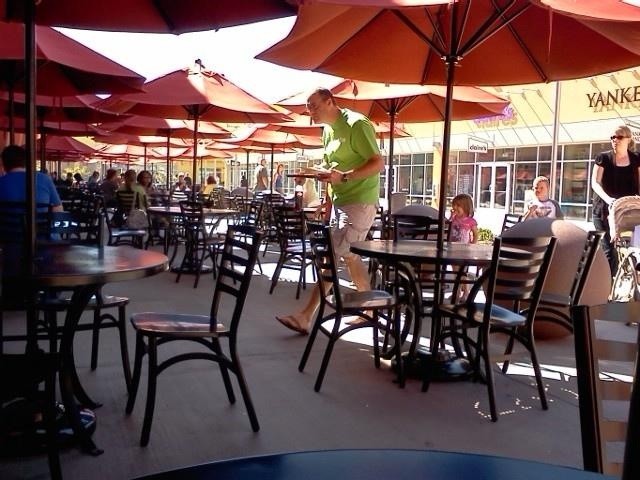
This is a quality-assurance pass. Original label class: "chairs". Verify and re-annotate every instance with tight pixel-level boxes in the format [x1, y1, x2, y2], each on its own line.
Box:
[0, 177, 640, 479]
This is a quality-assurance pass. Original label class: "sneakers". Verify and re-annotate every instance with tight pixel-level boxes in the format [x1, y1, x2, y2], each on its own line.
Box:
[448, 292, 471, 303]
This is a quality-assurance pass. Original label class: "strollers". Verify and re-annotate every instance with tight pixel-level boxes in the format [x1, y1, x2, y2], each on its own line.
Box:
[608, 194, 640, 326]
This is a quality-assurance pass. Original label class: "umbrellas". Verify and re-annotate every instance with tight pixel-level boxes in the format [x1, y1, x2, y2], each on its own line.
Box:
[0, 0, 302, 451]
[253, 1, 640, 384]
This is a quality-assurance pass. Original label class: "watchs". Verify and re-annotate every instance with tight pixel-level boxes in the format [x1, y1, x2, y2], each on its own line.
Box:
[342, 173, 349, 182]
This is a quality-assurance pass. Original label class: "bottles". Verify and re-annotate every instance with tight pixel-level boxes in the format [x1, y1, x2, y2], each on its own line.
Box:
[293, 181, 304, 209]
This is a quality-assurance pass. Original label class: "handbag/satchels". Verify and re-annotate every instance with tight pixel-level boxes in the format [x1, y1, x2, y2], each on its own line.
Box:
[125, 192, 153, 230]
[607, 253, 640, 304]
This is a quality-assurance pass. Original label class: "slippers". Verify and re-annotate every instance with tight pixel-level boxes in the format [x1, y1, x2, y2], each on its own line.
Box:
[345, 316, 381, 326]
[275, 314, 310, 336]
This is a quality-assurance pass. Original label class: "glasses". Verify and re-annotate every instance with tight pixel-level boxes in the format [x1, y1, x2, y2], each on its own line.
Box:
[610, 135, 628, 141]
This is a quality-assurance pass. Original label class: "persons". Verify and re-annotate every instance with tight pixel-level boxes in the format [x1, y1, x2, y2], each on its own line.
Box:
[447, 193, 478, 303]
[275, 86, 385, 334]
[590, 124, 640, 283]
[522, 176, 564, 223]
